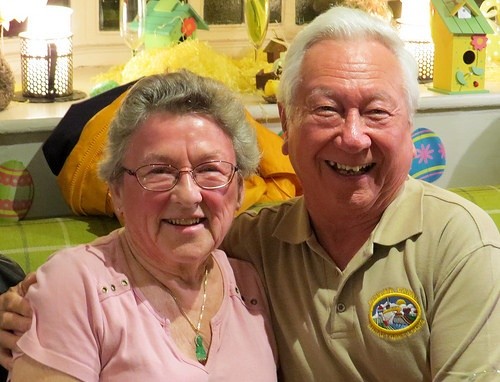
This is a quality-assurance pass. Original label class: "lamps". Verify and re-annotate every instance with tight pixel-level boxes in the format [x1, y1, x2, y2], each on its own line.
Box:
[12, 5, 87, 103]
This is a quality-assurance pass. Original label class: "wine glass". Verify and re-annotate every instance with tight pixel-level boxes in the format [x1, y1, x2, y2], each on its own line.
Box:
[119, 0, 146, 58]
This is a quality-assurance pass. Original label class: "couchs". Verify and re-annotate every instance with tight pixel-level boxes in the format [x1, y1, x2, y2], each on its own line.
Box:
[0, 184, 500, 275]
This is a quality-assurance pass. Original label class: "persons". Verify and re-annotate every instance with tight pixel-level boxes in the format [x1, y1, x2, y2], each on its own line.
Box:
[0, 0, 500, 382]
[138, 264, 210, 361]
[8, 70, 278, 382]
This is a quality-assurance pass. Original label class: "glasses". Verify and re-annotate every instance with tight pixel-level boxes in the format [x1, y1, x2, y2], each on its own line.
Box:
[121, 161, 238, 192]
